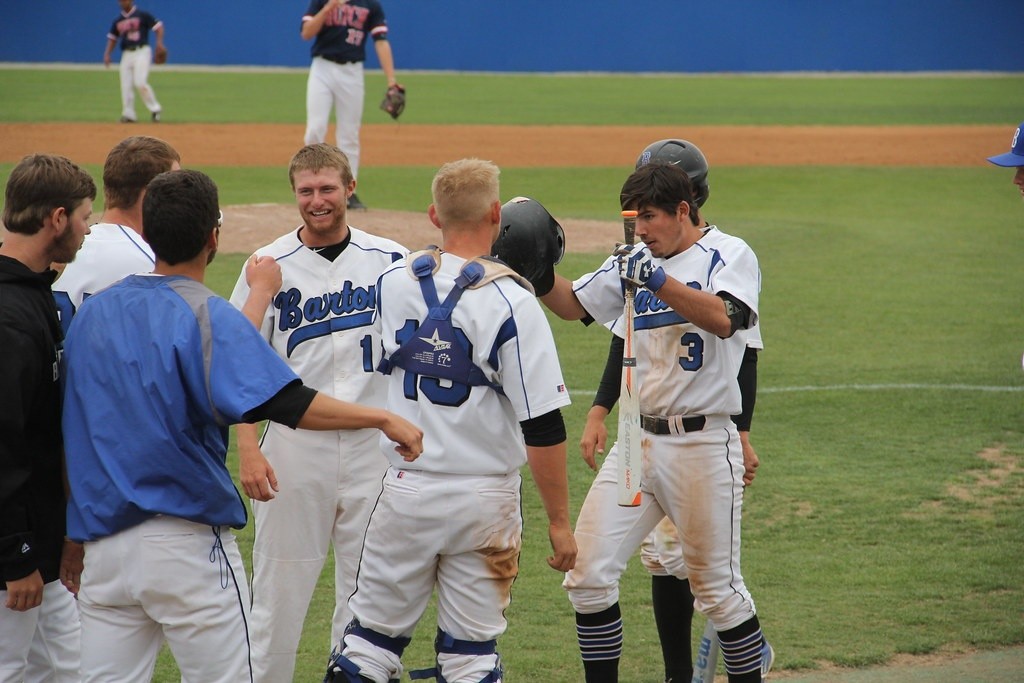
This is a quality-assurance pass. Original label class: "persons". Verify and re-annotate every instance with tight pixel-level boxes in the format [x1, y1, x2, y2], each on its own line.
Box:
[59, 170, 423, 683]
[51, 136, 180, 338]
[300, 0, 406, 211]
[103, 0, 167, 122]
[228, 142, 410, 683]
[323, 158, 578, 683]
[0, 154, 96, 683]
[492, 139, 775, 683]
[985, 124, 1024, 195]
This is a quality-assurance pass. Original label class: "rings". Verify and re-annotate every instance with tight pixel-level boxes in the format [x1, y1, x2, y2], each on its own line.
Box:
[67, 579, 73, 583]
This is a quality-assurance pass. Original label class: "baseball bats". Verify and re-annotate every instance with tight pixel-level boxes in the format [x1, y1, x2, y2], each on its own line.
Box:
[616, 210, 642, 507]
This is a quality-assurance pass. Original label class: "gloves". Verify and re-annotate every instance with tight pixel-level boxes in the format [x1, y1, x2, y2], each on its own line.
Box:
[612, 242, 666, 293]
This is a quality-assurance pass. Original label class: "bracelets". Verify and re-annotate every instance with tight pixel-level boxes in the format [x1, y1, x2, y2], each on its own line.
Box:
[64, 536, 71, 540]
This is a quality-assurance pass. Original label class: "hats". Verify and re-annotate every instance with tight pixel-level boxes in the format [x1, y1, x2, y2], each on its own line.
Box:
[986, 123, 1024, 167]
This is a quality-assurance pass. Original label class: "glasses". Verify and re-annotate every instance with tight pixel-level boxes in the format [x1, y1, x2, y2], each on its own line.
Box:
[217, 210, 223, 228]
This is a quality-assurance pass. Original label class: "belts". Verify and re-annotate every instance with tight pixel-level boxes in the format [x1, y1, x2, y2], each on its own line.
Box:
[640, 415, 706, 434]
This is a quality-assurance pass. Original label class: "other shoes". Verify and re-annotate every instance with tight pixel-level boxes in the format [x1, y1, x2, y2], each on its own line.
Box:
[152, 111, 160, 121]
[760, 636, 775, 683]
[347, 193, 366, 212]
[121, 116, 133, 122]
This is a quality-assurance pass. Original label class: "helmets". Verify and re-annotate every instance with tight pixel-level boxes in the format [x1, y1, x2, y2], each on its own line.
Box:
[635, 139, 709, 210]
[490, 197, 565, 297]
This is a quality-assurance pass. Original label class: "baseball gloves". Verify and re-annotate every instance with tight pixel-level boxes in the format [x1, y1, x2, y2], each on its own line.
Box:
[379, 82, 406, 120]
[154, 47, 167, 64]
